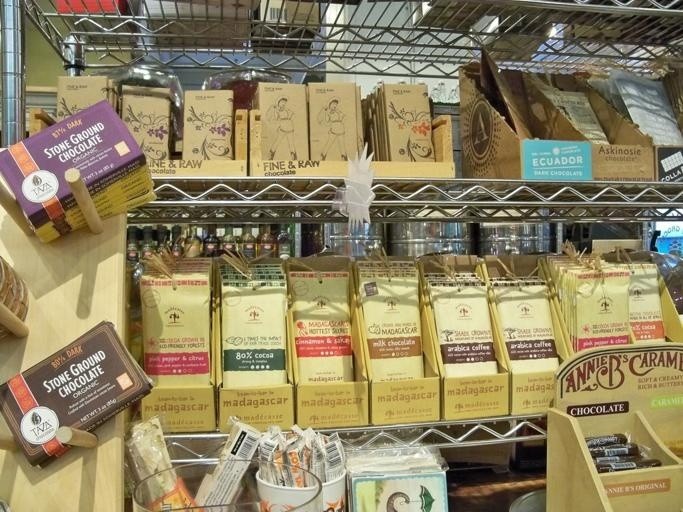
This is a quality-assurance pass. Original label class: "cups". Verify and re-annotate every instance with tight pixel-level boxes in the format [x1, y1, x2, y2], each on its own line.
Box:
[132, 460, 347, 511]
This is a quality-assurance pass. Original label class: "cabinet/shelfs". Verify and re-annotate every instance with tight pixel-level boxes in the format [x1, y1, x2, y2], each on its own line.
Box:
[20, 1, 683, 468]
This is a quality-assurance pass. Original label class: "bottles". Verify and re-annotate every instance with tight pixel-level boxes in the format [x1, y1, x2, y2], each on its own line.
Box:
[125, 221, 293, 318]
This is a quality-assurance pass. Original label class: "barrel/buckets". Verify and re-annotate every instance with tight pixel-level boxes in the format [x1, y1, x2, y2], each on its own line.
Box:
[306, 219, 551, 254]
